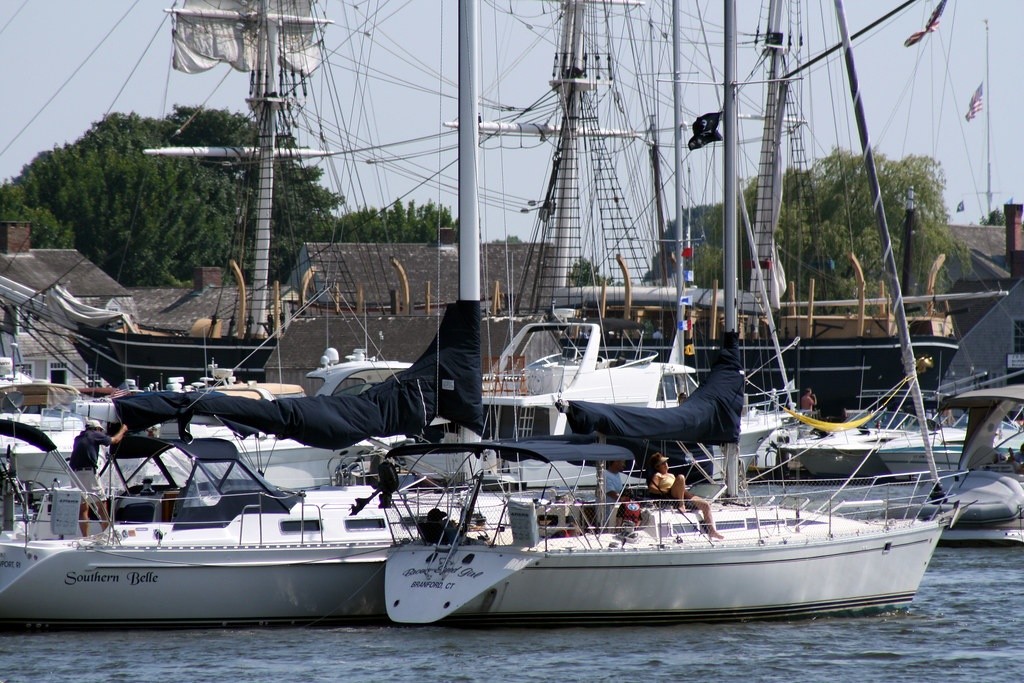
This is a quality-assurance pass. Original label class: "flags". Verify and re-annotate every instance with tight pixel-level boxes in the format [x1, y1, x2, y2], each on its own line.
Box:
[956, 201, 964, 212]
[677, 319, 691, 331]
[689, 111, 723, 150]
[966, 82, 983, 122]
[904, 0, 947, 48]
[683, 270, 693, 282]
[679, 295, 693, 305]
[682, 248, 693, 257]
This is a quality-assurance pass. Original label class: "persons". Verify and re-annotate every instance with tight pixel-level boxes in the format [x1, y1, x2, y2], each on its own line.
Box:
[426, 509, 492, 545]
[801, 388, 817, 411]
[648, 451, 724, 540]
[69, 420, 127, 538]
[943, 410, 955, 427]
[605, 460, 642, 533]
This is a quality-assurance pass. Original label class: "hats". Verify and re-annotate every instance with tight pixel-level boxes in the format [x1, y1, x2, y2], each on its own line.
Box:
[427, 509, 447, 521]
[650, 452, 669, 468]
[86, 419, 106, 431]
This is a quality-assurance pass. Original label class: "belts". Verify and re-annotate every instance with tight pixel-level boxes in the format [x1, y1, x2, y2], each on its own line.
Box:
[72, 467, 92, 471]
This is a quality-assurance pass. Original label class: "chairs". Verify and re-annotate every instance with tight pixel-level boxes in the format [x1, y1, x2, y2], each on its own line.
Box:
[418, 522, 449, 544]
[646, 453, 678, 486]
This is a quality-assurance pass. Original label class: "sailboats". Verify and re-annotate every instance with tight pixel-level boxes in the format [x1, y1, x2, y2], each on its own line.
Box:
[0, 0, 1023, 637]
